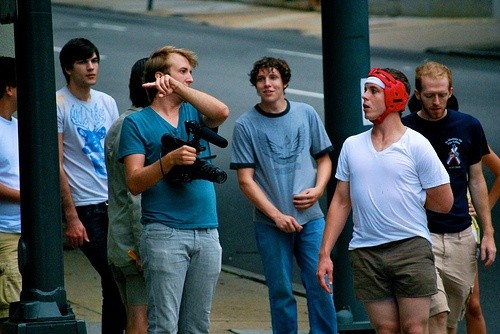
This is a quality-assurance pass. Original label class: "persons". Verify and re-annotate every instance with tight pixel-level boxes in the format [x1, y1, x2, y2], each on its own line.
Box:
[56, 38, 127, 334]
[230, 56, 338, 334]
[118, 46, 229, 334]
[104, 58, 148, 334]
[407, 91, 500, 334]
[316, 68, 454, 334]
[400, 61, 497, 334]
[0, 56, 22, 318]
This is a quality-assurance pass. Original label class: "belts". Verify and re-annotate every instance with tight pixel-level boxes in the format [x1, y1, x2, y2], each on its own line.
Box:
[76, 200, 108, 216]
